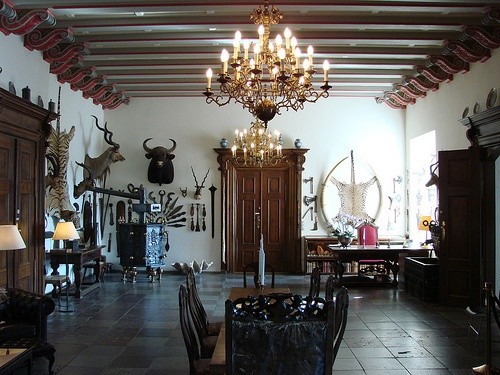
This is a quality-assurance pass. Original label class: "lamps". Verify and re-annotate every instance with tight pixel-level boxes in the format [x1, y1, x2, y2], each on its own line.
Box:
[53, 221, 81, 312]
[231, 118, 284, 168]
[201, 0, 333, 127]
[0, 225, 27, 289]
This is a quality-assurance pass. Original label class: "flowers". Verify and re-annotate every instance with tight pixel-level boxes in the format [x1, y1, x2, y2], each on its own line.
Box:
[327, 212, 364, 236]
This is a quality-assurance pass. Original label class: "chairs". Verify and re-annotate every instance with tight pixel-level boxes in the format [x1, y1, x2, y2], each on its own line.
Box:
[356, 221, 385, 279]
[178, 266, 349, 375]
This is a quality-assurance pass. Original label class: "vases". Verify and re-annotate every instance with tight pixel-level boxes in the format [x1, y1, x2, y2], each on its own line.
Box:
[338, 235, 354, 247]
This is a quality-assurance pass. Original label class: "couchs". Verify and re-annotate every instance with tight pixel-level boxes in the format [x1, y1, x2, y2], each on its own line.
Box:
[0, 288, 56, 375]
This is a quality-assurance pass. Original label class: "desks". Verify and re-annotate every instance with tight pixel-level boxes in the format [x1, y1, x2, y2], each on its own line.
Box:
[327, 244, 434, 290]
[0, 342, 37, 375]
[51, 245, 107, 299]
[210, 287, 291, 365]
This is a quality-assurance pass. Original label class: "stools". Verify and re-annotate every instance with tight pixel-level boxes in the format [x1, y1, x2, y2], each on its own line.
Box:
[305, 255, 334, 297]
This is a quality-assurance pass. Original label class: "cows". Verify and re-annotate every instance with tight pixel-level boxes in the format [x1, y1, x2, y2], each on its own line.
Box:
[143, 137, 177, 188]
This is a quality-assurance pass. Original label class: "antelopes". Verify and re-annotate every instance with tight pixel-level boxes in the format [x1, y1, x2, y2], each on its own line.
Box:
[83, 115, 126, 183]
[73, 161, 97, 199]
